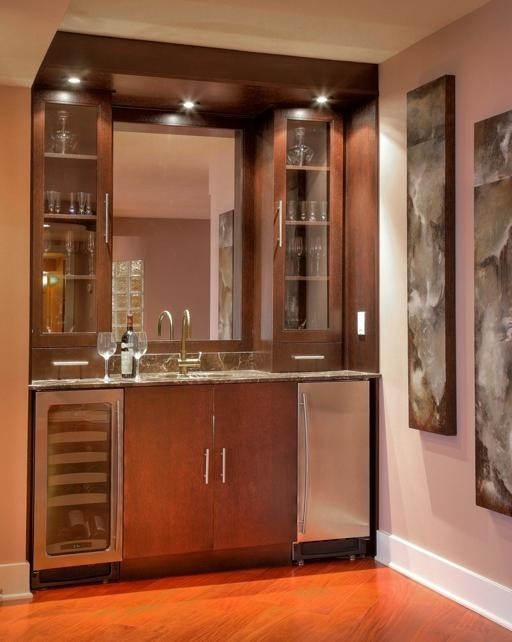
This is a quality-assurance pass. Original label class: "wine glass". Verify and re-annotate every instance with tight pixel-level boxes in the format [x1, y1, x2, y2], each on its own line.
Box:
[97, 331, 117, 382]
[45, 229, 97, 275]
[287, 235, 325, 276]
[128, 331, 148, 380]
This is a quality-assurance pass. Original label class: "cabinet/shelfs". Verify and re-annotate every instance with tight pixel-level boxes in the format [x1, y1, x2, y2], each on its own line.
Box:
[121, 381, 297, 578]
[253, 107, 344, 373]
[30, 88, 113, 380]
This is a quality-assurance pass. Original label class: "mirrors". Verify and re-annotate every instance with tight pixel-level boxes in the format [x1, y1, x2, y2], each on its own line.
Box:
[112, 108, 253, 351]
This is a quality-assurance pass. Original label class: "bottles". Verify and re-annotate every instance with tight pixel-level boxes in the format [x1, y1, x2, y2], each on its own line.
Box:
[286, 126, 315, 165]
[47, 109, 79, 153]
[120, 314, 136, 378]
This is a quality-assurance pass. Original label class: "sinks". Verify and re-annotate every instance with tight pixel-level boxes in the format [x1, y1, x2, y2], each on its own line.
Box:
[159, 373, 233, 378]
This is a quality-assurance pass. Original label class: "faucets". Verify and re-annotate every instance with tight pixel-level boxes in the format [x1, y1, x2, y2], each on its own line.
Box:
[178, 309, 203, 375]
[157, 311, 175, 341]
